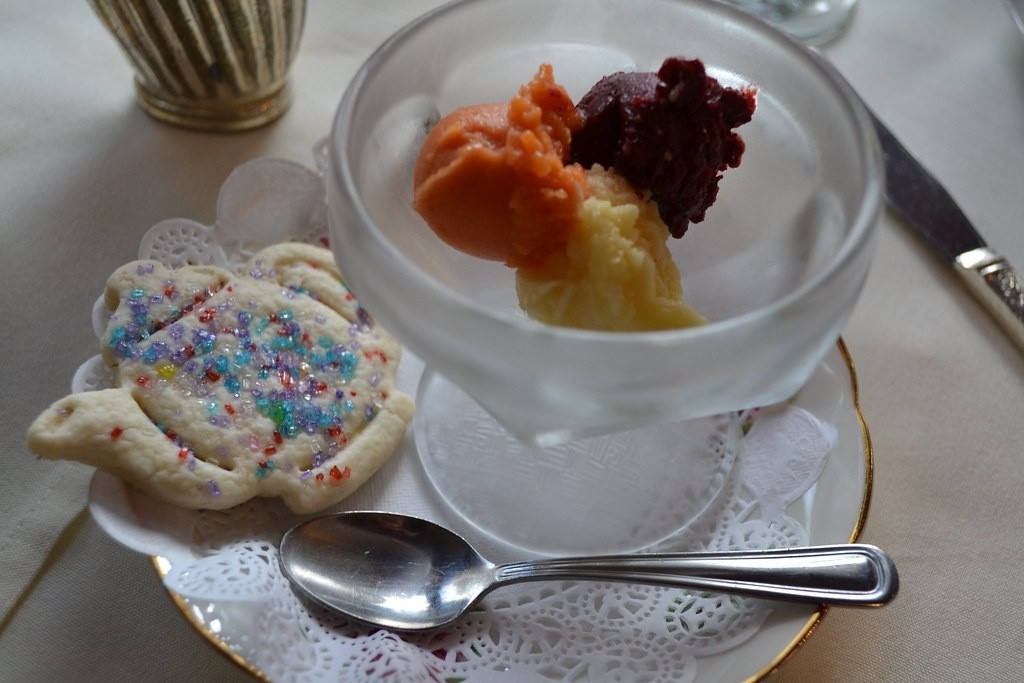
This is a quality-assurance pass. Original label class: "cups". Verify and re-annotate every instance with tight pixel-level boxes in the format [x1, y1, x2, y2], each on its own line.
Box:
[87, 1, 309, 131]
[726, 1, 855, 49]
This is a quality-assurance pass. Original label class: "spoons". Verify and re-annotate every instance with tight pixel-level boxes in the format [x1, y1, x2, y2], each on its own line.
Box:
[280, 513, 900, 632]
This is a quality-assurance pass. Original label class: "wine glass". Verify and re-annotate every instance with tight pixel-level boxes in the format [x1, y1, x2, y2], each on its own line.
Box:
[335, 3, 884, 568]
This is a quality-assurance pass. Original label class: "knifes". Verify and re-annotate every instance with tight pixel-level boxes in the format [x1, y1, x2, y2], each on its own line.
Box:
[875, 110, 1024, 356]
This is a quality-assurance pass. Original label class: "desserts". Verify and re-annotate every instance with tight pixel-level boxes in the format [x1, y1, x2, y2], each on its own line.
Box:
[415, 58, 757, 331]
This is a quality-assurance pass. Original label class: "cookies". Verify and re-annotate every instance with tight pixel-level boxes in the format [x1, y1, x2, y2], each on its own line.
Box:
[28, 245, 413, 514]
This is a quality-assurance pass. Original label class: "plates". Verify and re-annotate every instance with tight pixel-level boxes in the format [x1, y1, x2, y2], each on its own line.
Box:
[147, 319, 873, 683]
